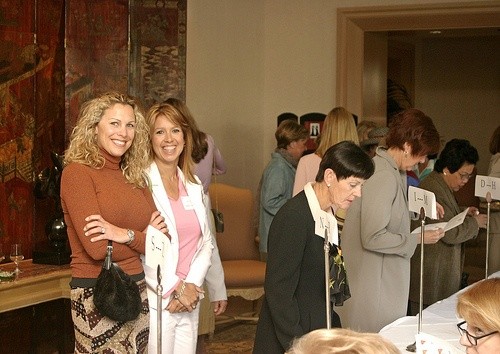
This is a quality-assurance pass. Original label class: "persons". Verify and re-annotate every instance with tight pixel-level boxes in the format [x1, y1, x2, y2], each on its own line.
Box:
[165, 98, 226, 191]
[61, 92, 227, 354]
[254, 108, 445, 354]
[456, 275, 500, 354]
[478, 125, 500, 275]
[407, 139, 488, 316]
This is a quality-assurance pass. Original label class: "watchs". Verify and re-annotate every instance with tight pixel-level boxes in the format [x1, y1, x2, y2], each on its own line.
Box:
[125, 228, 135, 244]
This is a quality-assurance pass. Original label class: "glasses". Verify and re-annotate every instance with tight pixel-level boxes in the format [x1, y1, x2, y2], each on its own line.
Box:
[417, 155, 430, 164]
[456, 172, 472, 179]
[457, 321, 500, 347]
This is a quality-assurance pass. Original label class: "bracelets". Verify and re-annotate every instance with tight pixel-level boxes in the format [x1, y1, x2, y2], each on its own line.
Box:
[174, 281, 186, 299]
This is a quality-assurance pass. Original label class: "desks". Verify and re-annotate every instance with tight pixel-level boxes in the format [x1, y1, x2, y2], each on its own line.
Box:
[0, 258, 71, 314]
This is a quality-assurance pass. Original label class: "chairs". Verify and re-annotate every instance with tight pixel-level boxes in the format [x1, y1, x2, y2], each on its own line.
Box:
[209, 183, 267, 327]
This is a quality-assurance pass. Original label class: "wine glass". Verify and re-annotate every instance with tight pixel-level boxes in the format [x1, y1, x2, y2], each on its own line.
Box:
[9, 244, 25, 274]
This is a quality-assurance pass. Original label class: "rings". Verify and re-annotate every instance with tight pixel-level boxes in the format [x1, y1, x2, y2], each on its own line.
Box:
[101, 227, 106, 233]
[222, 310, 225, 313]
[192, 305, 195, 309]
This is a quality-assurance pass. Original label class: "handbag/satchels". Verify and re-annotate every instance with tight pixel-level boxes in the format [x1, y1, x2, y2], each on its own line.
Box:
[93, 239, 142, 322]
[211, 208, 225, 233]
